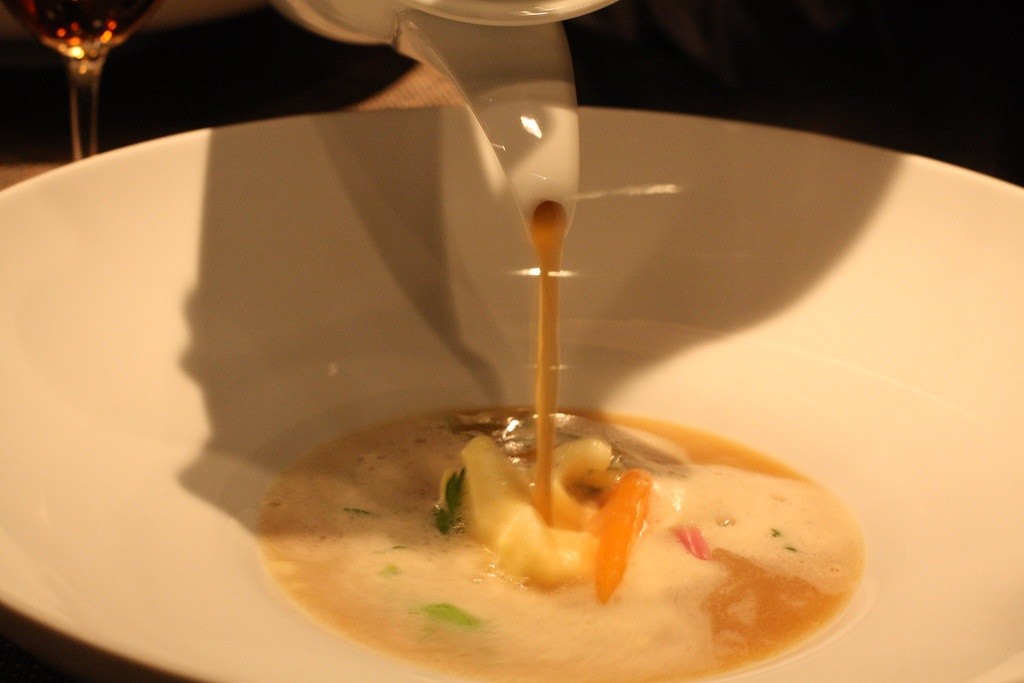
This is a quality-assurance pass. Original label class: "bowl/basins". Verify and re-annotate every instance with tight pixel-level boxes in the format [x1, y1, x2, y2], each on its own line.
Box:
[0, 107, 1024, 683]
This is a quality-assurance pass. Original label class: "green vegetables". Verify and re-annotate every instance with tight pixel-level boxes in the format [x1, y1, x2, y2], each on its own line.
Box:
[345, 467, 492, 628]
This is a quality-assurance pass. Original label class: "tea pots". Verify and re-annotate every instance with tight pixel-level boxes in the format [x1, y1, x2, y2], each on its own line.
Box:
[276, 0, 619, 244]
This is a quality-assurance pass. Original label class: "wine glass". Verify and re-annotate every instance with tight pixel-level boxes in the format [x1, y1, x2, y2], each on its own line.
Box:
[1, 0, 162, 164]
